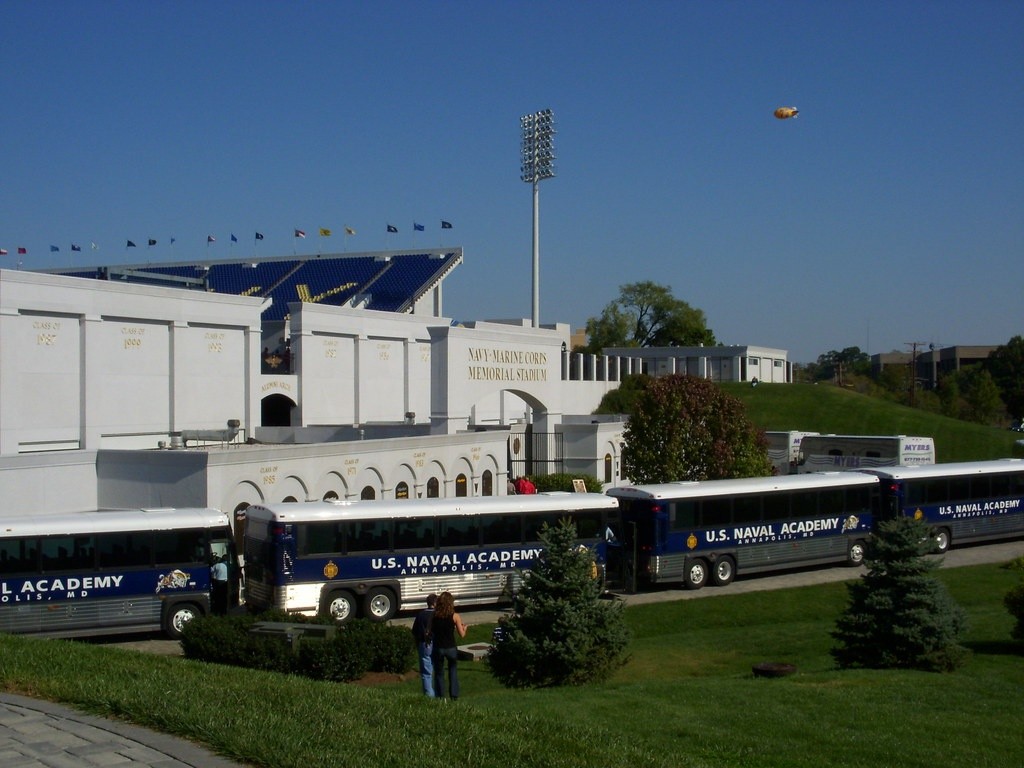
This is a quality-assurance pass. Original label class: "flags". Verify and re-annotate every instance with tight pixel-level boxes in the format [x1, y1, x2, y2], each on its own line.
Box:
[51, 245, 59, 252]
[414, 223, 425, 231]
[232, 235, 237, 241]
[345, 227, 355, 234]
[256, 233, 263, 240]
[127, 240, 136, 246]
[442, 221, 452, 228]
[148, 239, 157, 245]
[208, 236, 215, 241]
[171, 238, 175, 244]
[18, 248, 27, 254]
[0, 249, 7, 255]
[92, 241, 96, 248]
[71, 244, 80, 251]
[387, 225, 397, 232]
[320, 229, 330, 236]
[295, 230, 306, 238]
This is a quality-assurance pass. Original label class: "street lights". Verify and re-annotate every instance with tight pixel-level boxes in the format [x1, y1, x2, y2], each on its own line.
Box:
[519, 107, 557, 329]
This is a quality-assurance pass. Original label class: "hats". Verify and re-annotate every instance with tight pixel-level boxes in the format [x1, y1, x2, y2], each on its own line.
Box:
[427, 594, 441, 606]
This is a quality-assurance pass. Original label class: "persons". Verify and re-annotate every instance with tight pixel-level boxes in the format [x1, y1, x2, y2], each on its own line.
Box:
[491, 617, 508, 645]
[752, 377, 758, 387]
[411, 594, 437, 697]
[425, 592, 468, 701]
[211, 557, 227, 614]
[507, 476, 535, 495]
[262, 347, 290, 366]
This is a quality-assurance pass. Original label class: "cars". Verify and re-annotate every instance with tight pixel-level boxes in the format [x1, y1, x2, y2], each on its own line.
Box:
[1006, 420, 1024, 433]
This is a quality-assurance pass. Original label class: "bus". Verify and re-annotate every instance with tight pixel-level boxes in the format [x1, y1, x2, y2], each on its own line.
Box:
[241, 490, 620, 625]
[605, 470, 884, 591]
[0, 505, 243, 639]
[765, 431, 820, 477]
[796, 433, 936, 475]
[853, 457, 1024, 557]
